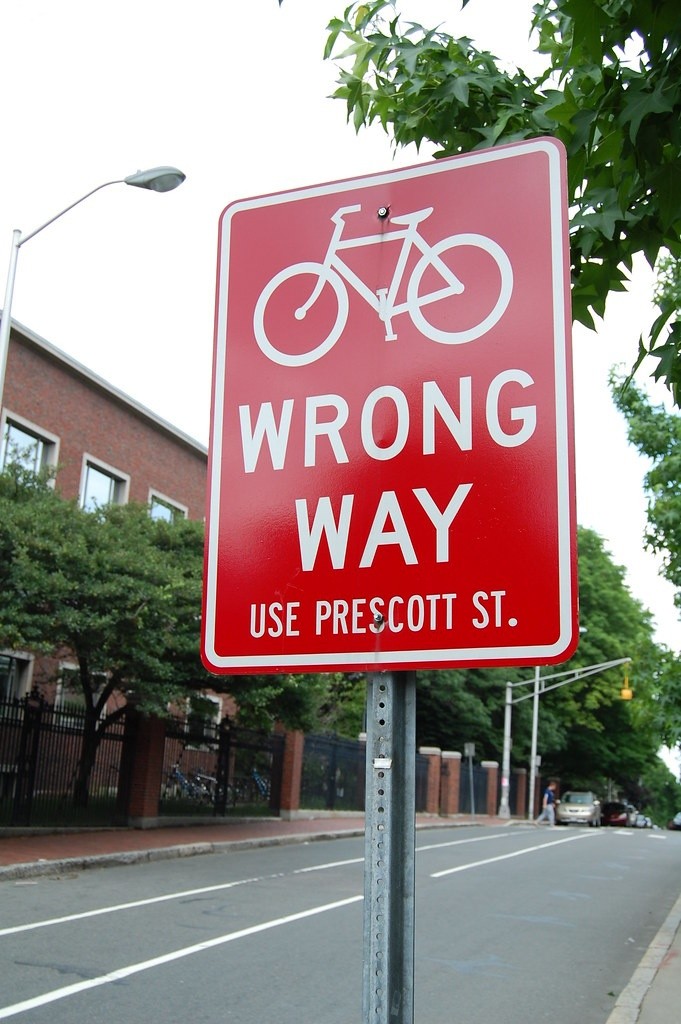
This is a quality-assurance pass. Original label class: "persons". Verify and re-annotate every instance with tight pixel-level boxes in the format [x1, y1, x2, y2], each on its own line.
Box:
[532, 782, 559, 830]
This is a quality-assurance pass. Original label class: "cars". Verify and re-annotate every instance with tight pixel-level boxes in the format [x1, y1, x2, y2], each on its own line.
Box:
[554, 791, 654, 829]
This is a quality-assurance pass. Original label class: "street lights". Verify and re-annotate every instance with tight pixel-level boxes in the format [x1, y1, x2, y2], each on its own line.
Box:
[498, 657, 632, 818]
[2, 165, 188, 399]
